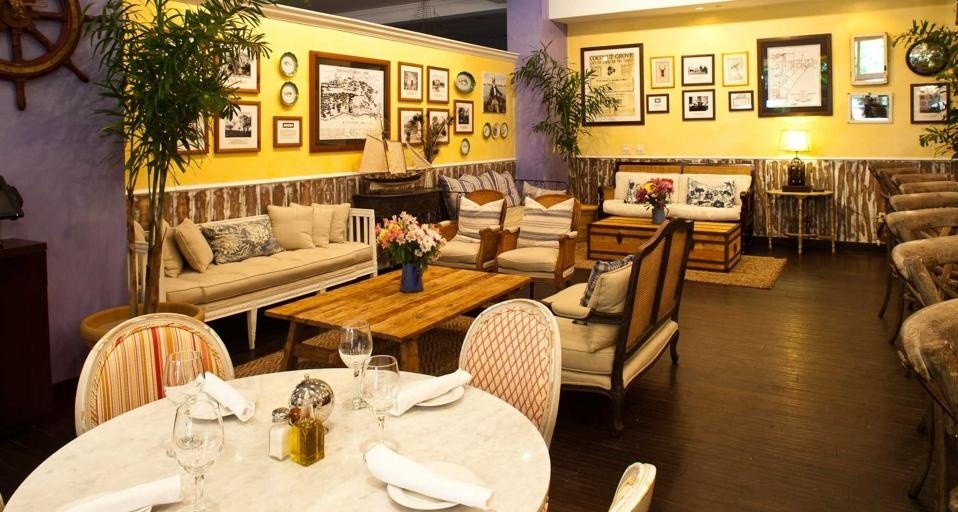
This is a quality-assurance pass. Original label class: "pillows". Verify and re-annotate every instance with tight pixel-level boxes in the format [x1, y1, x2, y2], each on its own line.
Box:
[572, 259, 632, 353]
[441, 171, 567, 210]
[624, 180, 639, 204]
[133, 201, 352, 278]
[687, 178, 735, 208]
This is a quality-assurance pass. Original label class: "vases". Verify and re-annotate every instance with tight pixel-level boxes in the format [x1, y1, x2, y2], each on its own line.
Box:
[652, 209, 665, 224]
[399, 261, 425, 294]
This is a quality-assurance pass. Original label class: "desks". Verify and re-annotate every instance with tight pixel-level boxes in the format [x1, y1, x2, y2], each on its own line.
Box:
[767, 190, 835, 254]
[588, 217, 743, 272]
[269, 265, 532, 372]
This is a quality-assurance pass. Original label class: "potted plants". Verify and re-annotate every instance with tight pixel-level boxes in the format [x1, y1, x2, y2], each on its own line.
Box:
[80, 0, 267, 351]
[509, 38, 620, 241]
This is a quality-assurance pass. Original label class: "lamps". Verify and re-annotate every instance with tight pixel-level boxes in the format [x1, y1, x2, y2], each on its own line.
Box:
[778, 130, 813, 192]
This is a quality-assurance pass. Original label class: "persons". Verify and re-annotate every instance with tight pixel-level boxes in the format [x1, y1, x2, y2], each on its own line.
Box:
[490, 80, 502, 113]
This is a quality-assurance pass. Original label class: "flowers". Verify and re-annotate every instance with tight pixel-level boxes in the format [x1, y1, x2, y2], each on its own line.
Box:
[376, 212, 445, 269]
[631, 177, 674, 208]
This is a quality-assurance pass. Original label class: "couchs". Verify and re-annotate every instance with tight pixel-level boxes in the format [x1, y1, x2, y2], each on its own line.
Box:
[599, 161, 753, 248]
[139, 208, 378, 350]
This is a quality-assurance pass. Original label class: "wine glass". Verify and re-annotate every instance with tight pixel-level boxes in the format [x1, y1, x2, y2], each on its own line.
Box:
[337, 319, 374, 410]
[172, 398, 225, 512]
[360, 354, 400, 462]
[162, 350, 206, 406]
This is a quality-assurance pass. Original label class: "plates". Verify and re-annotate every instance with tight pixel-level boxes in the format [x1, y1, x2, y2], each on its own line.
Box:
[387, 460, 461, 510]
[414, 385, 465, 408]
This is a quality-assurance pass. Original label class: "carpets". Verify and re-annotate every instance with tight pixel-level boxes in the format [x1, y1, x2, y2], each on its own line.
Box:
[573, 242, 788, 290]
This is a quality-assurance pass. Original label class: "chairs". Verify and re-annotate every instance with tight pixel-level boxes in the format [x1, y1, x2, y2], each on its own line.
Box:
[870, 161, 958, 510]
[535, 219, 694, 436]
[428, 190, 582, 288]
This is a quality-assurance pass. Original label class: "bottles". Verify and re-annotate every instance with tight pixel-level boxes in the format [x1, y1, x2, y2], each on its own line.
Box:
[289, 418, 325, 467]
[268, 408, 291, 461]
